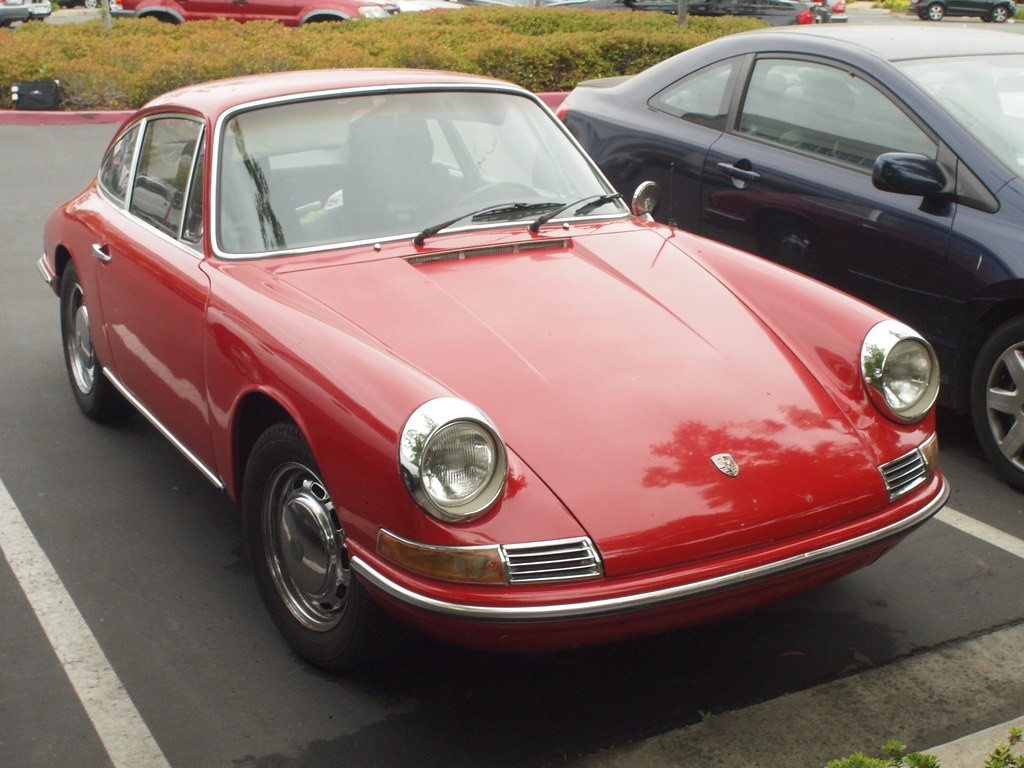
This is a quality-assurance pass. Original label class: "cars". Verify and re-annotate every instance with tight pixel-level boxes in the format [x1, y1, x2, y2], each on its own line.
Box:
[549, 22, 1024, 498]
[57, 0, 101, 9]
[99, 0, 850, 25]
[908, 0, 1018, 23]
[0, 0, 53, 27]
[38, 66, 949, 678]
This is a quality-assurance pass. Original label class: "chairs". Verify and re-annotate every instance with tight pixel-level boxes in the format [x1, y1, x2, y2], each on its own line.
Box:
[746, 64, 1014, 172]
[344, 113, 457, 236]
[178, 138, 306, 250]
